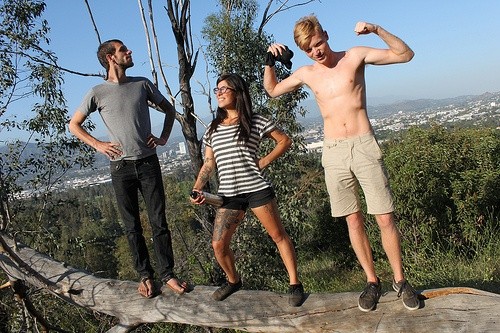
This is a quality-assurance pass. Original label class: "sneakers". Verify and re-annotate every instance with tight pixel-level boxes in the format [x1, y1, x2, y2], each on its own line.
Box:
[392, 278, 420, 311]
[287, 281, 304, 306]
[211, 278, 242, 302]
[358, 277, 381, 312]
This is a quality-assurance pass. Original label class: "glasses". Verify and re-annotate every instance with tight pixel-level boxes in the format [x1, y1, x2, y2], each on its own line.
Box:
[213, 87, 236, 95]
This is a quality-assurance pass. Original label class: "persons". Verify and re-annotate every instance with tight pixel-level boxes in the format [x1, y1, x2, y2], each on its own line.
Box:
[264, 16, 421, 311]
[68, 39, 189, 298]
[190, 73, 304, 307]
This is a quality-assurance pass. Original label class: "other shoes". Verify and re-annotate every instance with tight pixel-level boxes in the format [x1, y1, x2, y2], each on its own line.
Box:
[138, 277, 155, 299]
[161, 272, 189, 294]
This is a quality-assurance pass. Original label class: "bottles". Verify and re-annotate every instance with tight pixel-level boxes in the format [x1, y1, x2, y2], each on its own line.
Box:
[190, 190, 224, 207]
